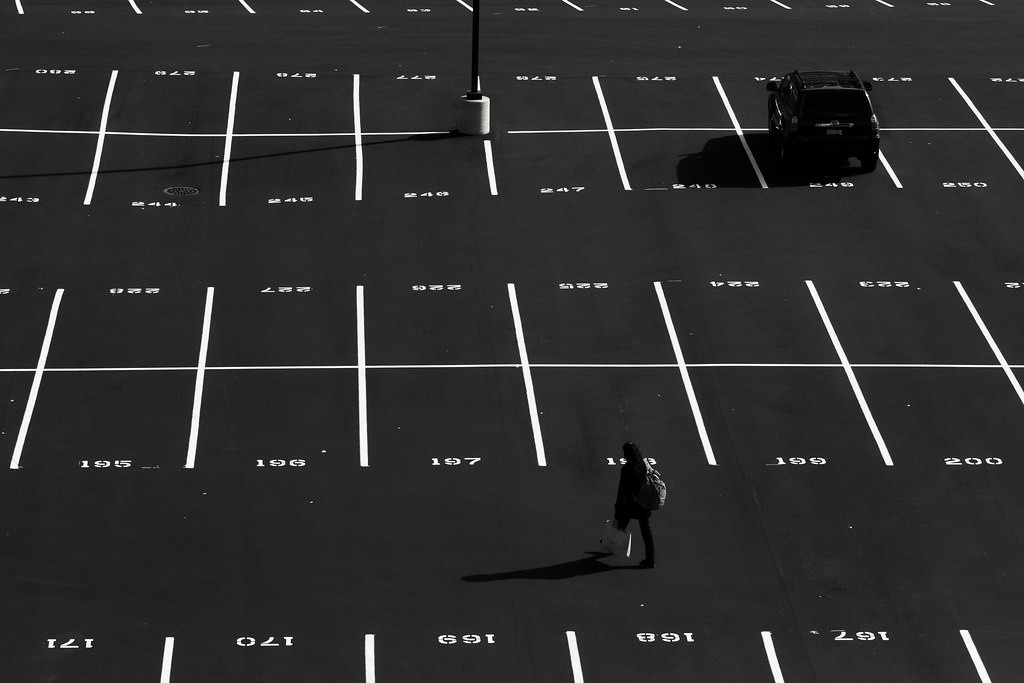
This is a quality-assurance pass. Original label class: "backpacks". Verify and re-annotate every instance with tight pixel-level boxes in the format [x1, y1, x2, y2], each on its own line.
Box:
[643, 457, 668, 510]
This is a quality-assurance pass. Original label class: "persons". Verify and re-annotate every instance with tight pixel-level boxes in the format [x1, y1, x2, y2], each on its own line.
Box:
[599, 440, 656, 570]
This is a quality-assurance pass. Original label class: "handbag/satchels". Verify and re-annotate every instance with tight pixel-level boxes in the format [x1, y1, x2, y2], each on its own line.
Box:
[601, 518, 632, 559]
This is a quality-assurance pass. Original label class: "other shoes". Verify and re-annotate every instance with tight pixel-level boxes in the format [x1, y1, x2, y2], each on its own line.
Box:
[599, 539, 603, 543]
[639, 559, 655, 569]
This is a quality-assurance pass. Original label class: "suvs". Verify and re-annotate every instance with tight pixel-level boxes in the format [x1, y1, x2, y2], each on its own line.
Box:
[766, 69, 881, 168]
